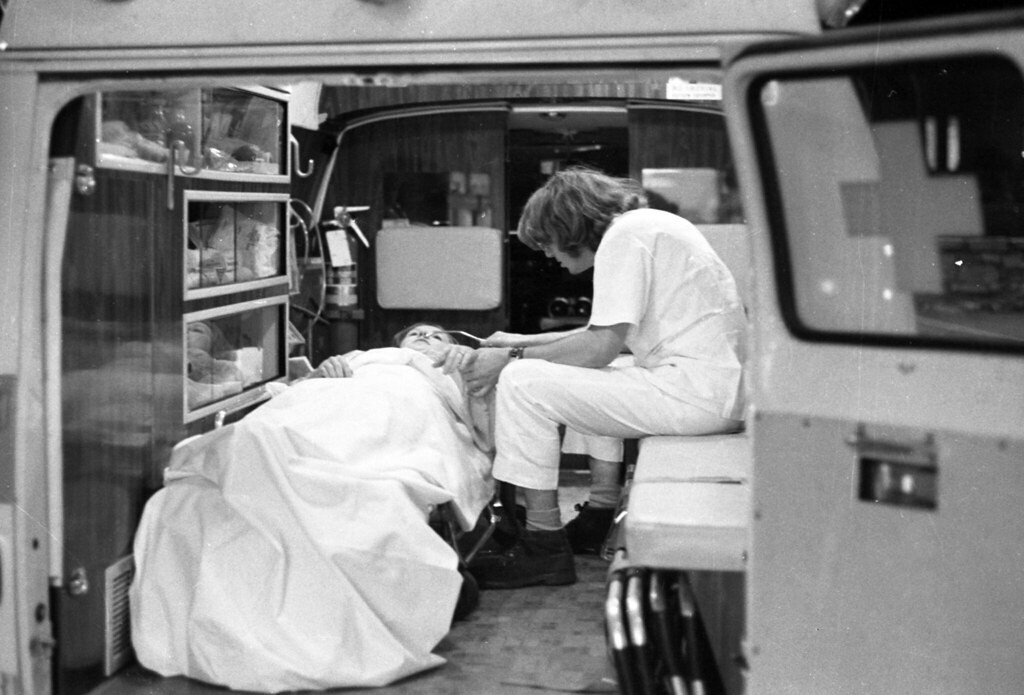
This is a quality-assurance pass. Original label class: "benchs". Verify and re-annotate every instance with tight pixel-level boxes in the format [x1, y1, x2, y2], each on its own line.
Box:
[625, 432, 748, 689]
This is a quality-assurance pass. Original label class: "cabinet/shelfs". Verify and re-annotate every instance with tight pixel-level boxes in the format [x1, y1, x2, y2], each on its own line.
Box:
[51, 190, 291, 695]
[89, 86, 293, 185]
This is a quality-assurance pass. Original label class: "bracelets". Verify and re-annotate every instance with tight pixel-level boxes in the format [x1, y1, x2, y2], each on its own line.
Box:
[508, 345, 524, 360]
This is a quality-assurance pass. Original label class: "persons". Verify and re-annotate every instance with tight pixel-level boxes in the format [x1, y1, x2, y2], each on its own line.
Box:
[65, 321, 240, 422]
[183, 322, 497, 504]
[466, 169, 751, 589]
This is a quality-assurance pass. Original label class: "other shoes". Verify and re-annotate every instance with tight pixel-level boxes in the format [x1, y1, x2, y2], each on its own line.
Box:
[565, 501, 616, 549]
[468, 527, 578, 589]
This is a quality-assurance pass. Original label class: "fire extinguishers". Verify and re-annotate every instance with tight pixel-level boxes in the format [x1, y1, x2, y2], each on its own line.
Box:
[316, 203, 371, 354]
